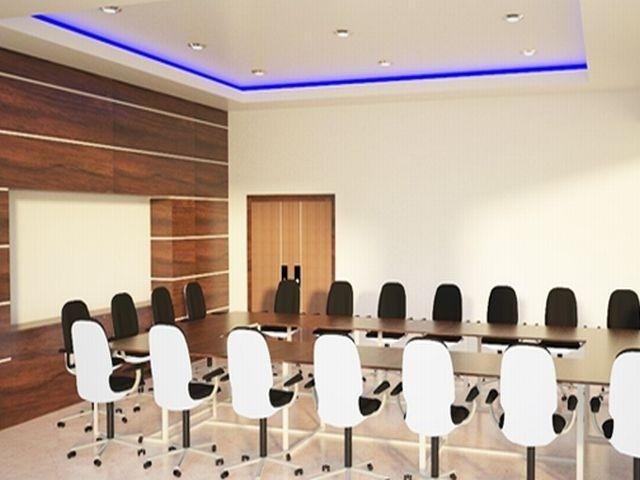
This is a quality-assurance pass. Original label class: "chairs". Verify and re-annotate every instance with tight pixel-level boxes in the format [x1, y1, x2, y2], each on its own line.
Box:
[543, 287, 582, 349]
[480, 285, 521, 354]
[486, 342, 579, 479]
[422, 282, 466, 346]
[597, 347, 640, 480]
[54, 280, 213, 439]
[65, 318, 146, 467]
[390, 336, 479, 478]
[142, 323, 225, 477]
[605, 288, 640, 330]
[365, 280, 407, 347]
[312, 279, 357, 345]
[260, 279, 304, 341]
[221, 326, 304, 478]
[304, 332, 390, 480]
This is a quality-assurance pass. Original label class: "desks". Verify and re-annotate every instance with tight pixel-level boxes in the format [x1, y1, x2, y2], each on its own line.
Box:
[105, 314, 638, 463]
